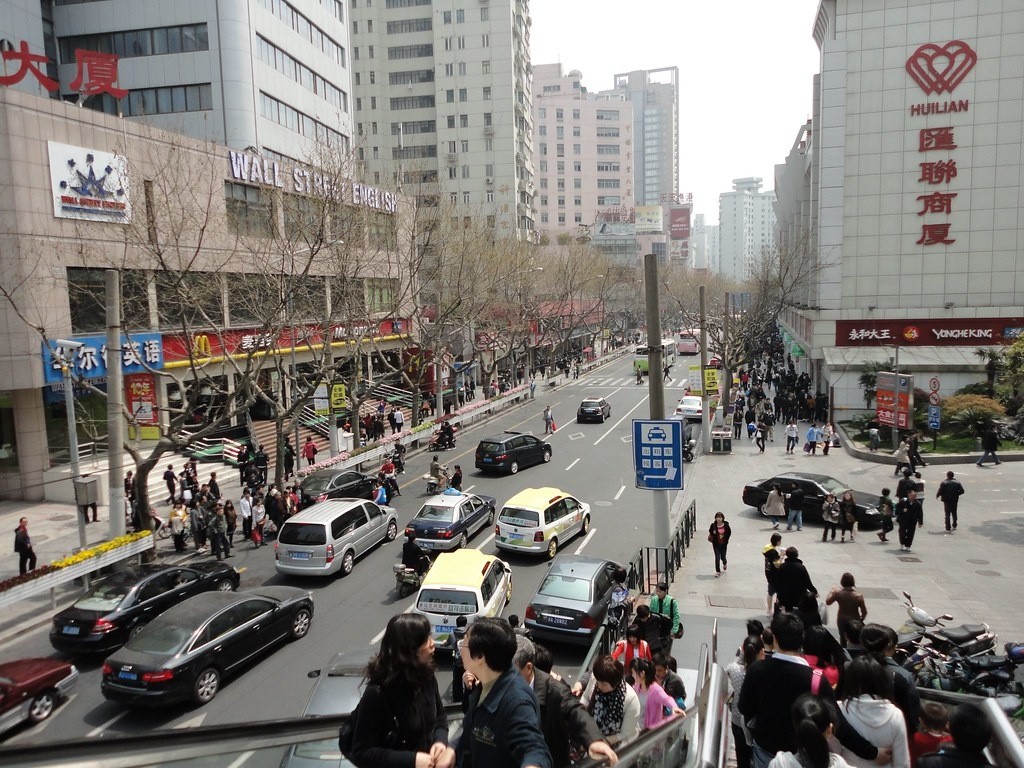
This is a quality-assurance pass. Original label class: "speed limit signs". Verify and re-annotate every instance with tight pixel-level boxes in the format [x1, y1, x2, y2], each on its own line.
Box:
[929, 378, 940, 392]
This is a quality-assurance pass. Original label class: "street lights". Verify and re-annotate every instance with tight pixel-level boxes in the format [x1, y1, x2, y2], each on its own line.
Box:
[273, 238, 345, 471]
[884, 341, 899, 454]
[517, 266, 544, 311]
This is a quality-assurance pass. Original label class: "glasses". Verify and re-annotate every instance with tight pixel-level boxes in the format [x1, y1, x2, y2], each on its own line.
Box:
[457, 640, 470, 654]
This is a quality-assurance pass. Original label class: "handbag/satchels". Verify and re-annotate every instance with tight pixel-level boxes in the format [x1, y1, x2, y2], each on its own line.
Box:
[338, 680, 407, 768]
[669, 598, 684, 638]
[757, 430, 762, 438]
[708, 523, 715, 542]
[804, 442, 811, 452]
[552, 421, 556, 431]
[251, 528, 261, 542]
[183, 489, 192, 500]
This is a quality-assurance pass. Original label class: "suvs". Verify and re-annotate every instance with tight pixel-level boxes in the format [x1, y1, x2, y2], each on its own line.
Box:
[409, 547, 514, 654]
[273, 498, 399, 585]
[494, 485, 591, 561]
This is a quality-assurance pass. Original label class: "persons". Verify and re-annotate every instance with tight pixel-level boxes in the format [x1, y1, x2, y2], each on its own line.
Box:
[775, 547, 820, 605]
[123, 436, 317, 559]
[708, 511, 732, 577]
[807, 422, 822, 455]
[663, 362, 671, 381]
[894, 430, 930, 475]
[337, 571, 1000, 768]
[781, 421, 799, 453]
[866, 416, 881, 452]
[733, 319, 829, 453]
[82, 475, 101, 524]
[762, 470, 966, 554]
[636, 365, 644, 385]
[483, 329, 640, 400]
[820, 421, 833, 455]
[762, 532, 785, 616]
[341, 370, 464, 506]
[14, 517, 37, 574]
[976, 424, 1001, 466]
[402, 533, 431, 572]
[543, 406, 556, 434]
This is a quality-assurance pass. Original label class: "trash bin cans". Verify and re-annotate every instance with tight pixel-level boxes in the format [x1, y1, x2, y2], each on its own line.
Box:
[71, 540, 112, 586]
[712, 431, 732, 454]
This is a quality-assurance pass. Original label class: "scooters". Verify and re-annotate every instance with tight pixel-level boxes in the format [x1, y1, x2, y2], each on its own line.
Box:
[420, 464, 455, 494]
[126, 508, 174, 539]
[393, 545, 435, 599]
[888, 592, 1024, 722]
[681, 438, 697, 462]
[427, 426, 458, 453]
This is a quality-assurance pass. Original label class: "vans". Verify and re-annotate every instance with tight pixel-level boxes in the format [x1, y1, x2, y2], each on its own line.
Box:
[474, 430, 553, 475]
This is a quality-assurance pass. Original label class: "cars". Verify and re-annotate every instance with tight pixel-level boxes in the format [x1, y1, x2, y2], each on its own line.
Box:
[683, 386, 694, 396]
[665, 414, 692, 441]
[100, 585, 317, 718]
[742, 471, 897, 531]
[49, 558, 241, 668]
[0, 654, 79, 741]
[278, 642, 383, 768]
[577, 396, 612, 423]
[676, 396, 703, 421]
[402, 488, 498, 556]
[709, 355, 722, 368]
[524, 551, 628, 646]
[296, 468, 381, 508]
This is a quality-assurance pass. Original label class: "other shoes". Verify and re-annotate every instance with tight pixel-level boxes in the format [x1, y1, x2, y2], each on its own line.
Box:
[176, 542, 237, 562]
[767, 610, 775, 617]
[723, 561, 727, 570]
[797, 527, 801, 531]
[821, 532, 913, 552]
[953, 523, 957, 528]
[715, 571, 720, 577]
[773, 522, 780, 530]
[946, 527, 950, 532]
[787, 526, 791, 530]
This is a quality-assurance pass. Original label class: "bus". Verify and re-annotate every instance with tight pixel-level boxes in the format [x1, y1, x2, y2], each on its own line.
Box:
[678, 328, 701, 356]
[633, 337, 680, 376]
